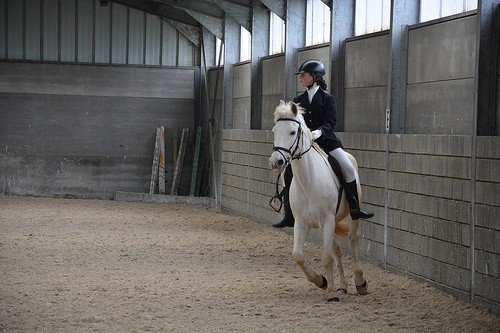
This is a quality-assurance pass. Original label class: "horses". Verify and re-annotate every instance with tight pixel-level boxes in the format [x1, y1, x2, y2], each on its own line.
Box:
[269, 100, 368, 302]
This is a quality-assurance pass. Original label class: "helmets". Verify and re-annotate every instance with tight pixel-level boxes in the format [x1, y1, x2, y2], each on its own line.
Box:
[294, 59, 325, 75]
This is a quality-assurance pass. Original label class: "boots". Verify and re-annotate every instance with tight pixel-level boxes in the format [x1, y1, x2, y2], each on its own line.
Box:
[345, 179, 374, 220]
[272, 186, 294, 228]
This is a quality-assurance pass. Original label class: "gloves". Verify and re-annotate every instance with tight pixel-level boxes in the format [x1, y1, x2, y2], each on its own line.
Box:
[312, 130, 323, 140]
